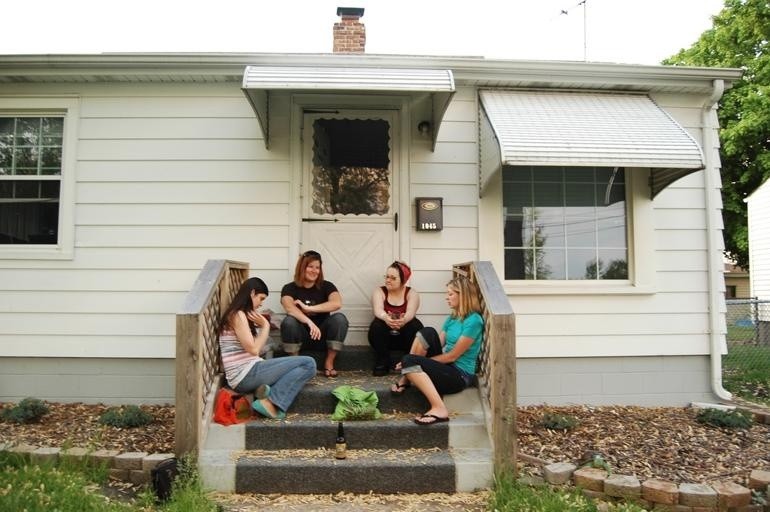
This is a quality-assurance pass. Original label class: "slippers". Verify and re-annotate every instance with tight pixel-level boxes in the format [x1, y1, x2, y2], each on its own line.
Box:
[324, 368, 338, 378]
[415, 413, 450, 425]
[392, 382, 406, 395]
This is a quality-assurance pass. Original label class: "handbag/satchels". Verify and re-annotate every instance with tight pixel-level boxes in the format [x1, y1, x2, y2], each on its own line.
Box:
[213, 388, 252, 426]
[331, 386, 381, 420]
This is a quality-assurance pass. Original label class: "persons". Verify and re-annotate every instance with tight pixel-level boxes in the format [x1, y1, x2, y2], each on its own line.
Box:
[389, 277, 485, 425]
[219, 277, 317, 419]
[368, 261, 420, 376]
[280, 251, 349, 377]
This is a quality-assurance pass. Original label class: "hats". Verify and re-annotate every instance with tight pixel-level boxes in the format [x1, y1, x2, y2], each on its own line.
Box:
[395, 261, 411, 284]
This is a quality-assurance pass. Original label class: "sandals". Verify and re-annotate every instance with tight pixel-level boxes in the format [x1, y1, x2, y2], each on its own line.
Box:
[255, 384, 271, 398]
[252, 399, 286, 420]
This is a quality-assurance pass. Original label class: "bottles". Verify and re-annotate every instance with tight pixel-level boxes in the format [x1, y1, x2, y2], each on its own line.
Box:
[335, 422, 347, 460]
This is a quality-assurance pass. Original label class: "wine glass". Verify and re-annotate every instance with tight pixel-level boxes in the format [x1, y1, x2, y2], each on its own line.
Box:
[391, 312, 401, 336]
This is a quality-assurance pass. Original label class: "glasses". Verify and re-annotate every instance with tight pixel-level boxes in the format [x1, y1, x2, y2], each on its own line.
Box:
[384, 274, 401, 281]
